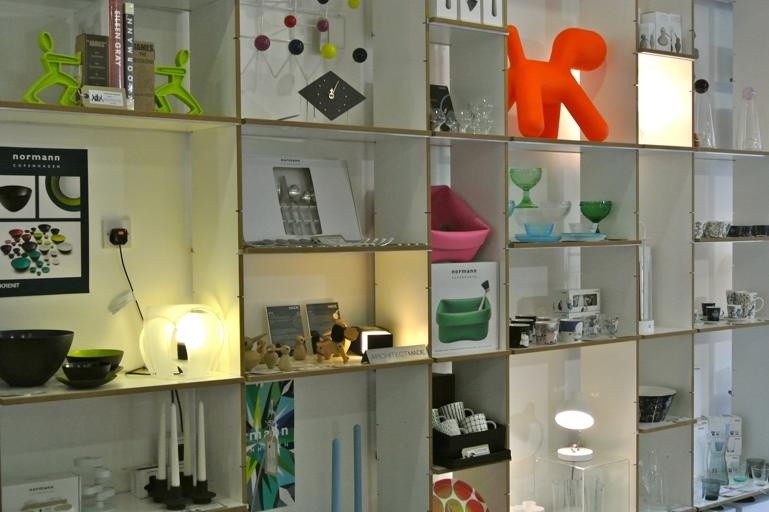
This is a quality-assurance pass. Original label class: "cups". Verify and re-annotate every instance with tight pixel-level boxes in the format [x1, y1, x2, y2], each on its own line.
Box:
[509, 309, 619, 348]
[432, 402, 496, 436]
[509, 500, 546, 511]
[728, 224, 769, 239]
[692, 221, 707, 240]
[726, 287, 765, 317]
[524, 222, 554, 235]
[704, 220, 731, 240]
[733, 456, 768, 484]
[508, 199, 515, 216]
[701, 301, 726, 321]
[702, 477, 721, 500]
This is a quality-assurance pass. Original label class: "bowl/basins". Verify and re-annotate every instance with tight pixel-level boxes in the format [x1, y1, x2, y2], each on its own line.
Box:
[0, 224, 72, 277]
[62, 360, 111, 378]
[639, 386, 679, 423]
[0, 329, 75, 385]
[66, 347, 124, 370]
[0, 185, 33, 212]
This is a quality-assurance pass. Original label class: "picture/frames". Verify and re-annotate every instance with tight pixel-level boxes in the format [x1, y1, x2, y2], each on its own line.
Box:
[261, 297, 345, 356]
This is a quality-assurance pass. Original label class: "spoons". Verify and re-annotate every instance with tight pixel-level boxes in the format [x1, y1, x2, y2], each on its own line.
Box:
[286, 183, 318, 235]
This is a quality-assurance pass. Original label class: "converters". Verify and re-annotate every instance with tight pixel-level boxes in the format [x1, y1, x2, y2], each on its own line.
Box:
[109, 227, 128, 245]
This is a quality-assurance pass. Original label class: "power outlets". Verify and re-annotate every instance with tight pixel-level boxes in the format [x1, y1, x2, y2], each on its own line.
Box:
[99, 217, 131, 249]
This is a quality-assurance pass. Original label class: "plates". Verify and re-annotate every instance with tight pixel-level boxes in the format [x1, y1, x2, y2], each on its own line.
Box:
[699, 314, 727, 325]
[560, 233, 608, 242]
[56, 375, 118, 389]
[728, 318, 763, 324]
[515, 233, 563, 242]
[44, 176, 80, 212]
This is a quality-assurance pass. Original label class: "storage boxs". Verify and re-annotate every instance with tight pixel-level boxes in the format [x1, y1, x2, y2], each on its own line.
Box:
[702, 413, 743, 456]
[703, 450, 741, 485]
[74, 33, 156, 113]
[429, 260, 500, 356]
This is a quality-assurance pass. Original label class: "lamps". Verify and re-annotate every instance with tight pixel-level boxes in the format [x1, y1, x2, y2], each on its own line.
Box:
[140, 300, 226, 384]
[550, 411, 595, 463]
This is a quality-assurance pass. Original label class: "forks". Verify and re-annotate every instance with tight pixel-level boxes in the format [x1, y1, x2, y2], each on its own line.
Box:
[319, 234, 394, 249]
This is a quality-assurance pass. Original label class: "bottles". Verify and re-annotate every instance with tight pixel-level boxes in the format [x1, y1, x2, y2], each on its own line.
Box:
[706, 434, 732, 485]
[739, 87, 762, 150]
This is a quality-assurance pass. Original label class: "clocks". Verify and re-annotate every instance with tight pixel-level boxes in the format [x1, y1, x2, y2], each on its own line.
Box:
[297, 71, 368, 124]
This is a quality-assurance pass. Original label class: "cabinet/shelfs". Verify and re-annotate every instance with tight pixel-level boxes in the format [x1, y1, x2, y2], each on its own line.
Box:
[0, 0, 769, 511]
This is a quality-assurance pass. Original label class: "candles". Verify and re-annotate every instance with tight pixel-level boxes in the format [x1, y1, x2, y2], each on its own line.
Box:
[169, 404, 181, 487]
[352, 422, 364, 511]
[156, 399, 167, 480]
[197, 398, 207, 482]
[331, 435, 341, 511]
[180, 409, 192, 476]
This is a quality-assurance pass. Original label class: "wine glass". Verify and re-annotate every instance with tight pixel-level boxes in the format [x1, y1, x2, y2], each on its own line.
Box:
[430, 94, 498, 134]
[510, 166, 543, 208]
[542, 200, 571, 231]
[577, 201, 612, 230]
